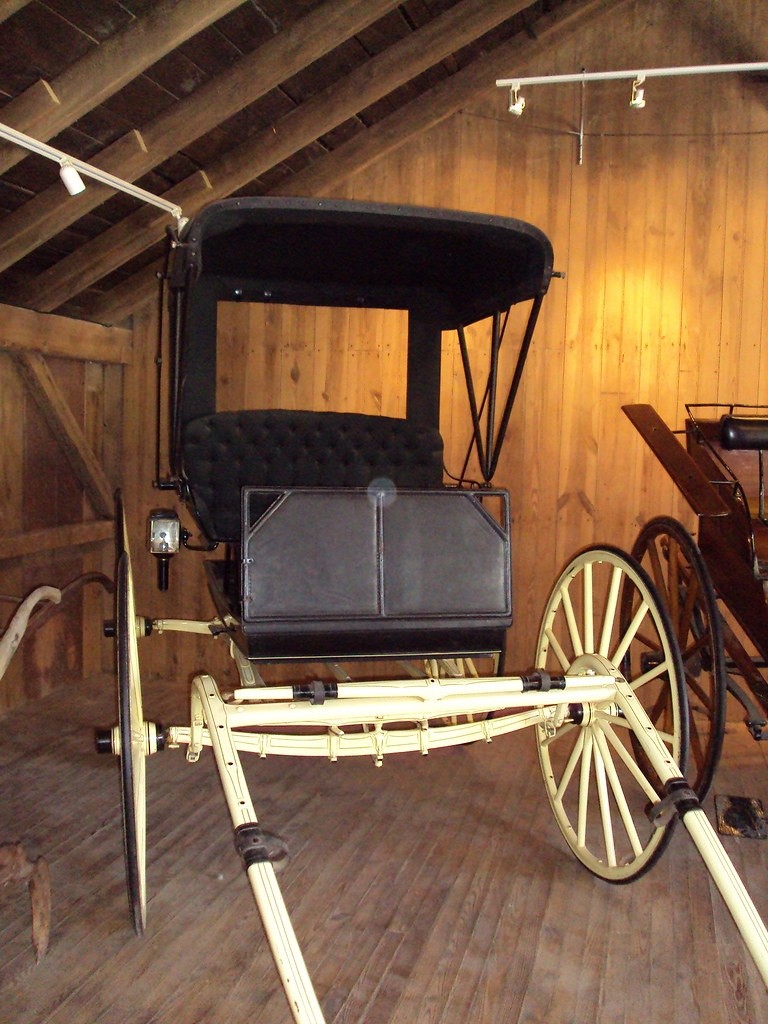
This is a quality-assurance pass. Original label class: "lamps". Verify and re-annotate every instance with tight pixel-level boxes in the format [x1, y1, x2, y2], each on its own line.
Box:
[495, 62, 768, 117]
[0, 123, 191, 237]
[145, 509, 219, 593]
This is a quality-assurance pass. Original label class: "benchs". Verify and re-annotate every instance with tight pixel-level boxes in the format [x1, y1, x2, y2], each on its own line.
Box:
[178, 407, 484, 540]
[719, 417, 768, 579]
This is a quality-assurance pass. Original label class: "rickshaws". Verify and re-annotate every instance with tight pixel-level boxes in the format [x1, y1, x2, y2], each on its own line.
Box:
[614, 401, 767, 811]
[91, 195, 767, 1024]
